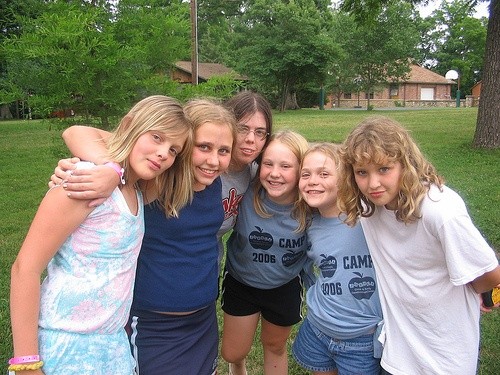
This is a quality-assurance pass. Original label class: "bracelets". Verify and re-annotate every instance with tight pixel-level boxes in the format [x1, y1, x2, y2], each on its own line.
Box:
[8, 355, 44, 371]
[105, 161, 125, 184]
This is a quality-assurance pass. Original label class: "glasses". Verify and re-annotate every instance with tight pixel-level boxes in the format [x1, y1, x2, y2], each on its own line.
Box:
[237, 127, 270, 138]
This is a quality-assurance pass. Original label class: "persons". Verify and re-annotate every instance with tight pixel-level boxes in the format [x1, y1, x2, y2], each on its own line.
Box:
[336, 117, 500, 375]
[47, 91, 383, 375]
[8, 95, 193, 375]
